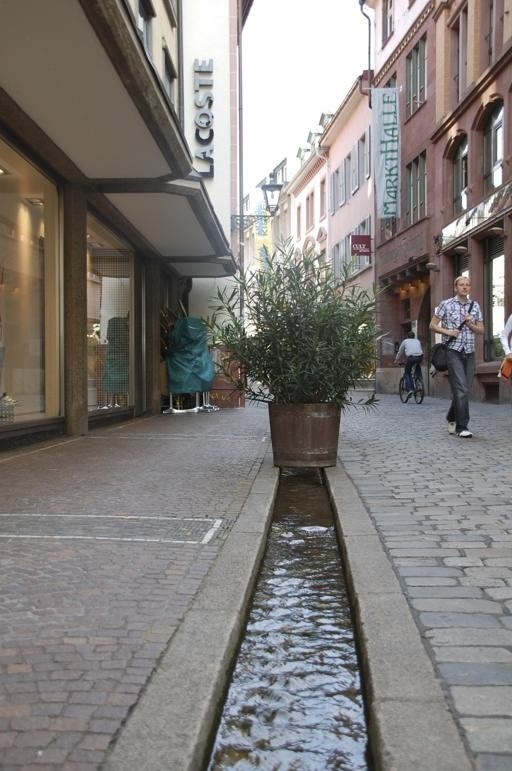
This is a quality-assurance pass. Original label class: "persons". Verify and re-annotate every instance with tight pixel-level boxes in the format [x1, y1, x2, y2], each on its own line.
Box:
[499, 314, 512, 362]
[428, 276, 483, 438]
[393, 332, 424, 397]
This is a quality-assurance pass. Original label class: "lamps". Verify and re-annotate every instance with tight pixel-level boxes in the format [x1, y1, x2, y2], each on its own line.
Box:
[232, 178, 283, 232]
[426, 227, 507, 272]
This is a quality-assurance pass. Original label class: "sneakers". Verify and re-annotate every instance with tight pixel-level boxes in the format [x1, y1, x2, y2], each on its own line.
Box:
[456, 429, 473, 438]
[407, 389, 414, 397]
[447, 420, 456, 434]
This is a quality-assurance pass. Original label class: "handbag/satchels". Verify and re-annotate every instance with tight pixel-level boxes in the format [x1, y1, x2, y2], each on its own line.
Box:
[429, 343, 449, 372]
[496, 357, 512, 382]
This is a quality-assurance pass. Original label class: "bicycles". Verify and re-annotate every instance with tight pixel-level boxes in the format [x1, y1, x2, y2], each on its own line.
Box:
[398, 357, 424, 404]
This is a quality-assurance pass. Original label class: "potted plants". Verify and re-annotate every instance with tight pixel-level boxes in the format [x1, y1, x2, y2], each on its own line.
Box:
[204, 236, 389, 468]
